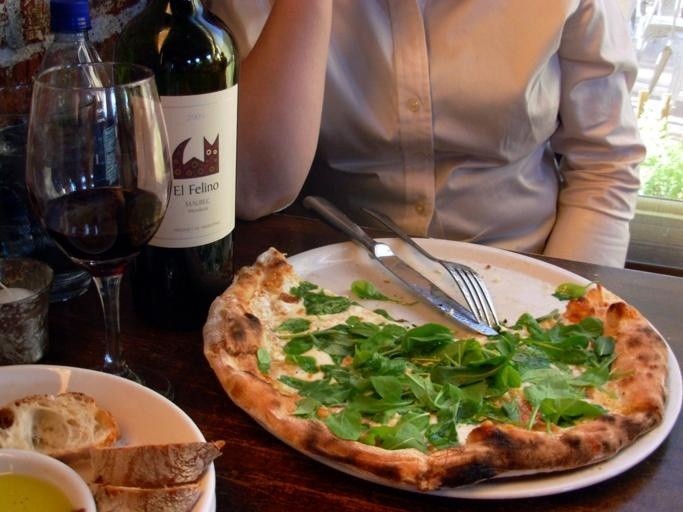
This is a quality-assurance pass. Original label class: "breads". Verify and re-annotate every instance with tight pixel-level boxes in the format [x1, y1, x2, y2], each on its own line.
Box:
[0, 391, 224, 512]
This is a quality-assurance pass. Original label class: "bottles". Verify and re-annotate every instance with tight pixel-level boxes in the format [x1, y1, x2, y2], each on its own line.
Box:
[24, 3, 118, 301]
[118, 1, 245, 333]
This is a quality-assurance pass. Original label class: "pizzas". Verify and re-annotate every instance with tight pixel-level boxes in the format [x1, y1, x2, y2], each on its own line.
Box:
[202, 246, 670, 492]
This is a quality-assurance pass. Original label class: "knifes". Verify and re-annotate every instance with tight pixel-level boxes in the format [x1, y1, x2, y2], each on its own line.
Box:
[299, 191, 500, 340]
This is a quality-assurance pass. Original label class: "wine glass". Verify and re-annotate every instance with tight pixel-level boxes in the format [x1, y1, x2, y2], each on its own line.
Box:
[20, 60, 176, 389]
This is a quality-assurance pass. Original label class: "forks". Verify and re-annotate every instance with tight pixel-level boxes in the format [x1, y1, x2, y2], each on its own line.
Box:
[362, 204, 502, 331]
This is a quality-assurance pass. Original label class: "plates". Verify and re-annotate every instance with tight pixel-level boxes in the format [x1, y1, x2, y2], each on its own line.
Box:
[249, 235, 682, 501]
[0, 448, 97, 512]
[0, 362, 216, 512]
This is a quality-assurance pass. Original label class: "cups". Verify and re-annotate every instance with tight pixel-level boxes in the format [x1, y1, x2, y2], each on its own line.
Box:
[0, 123, 93, 303]
[0, 255, 54, 363]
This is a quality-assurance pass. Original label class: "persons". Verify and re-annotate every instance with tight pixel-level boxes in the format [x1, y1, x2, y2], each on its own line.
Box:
[211, 1, 647, 271]
